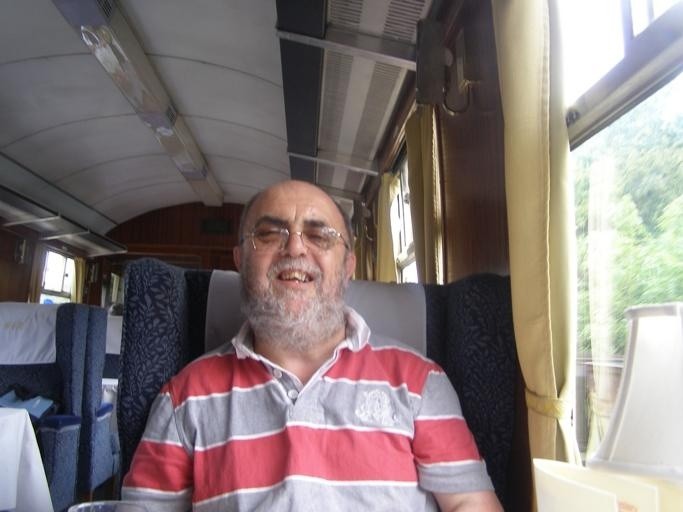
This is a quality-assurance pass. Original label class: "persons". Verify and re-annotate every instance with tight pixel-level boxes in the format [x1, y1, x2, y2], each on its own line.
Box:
[113, 177, 504, 511]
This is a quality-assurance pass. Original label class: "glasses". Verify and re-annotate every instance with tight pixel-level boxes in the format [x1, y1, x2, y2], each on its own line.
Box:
[238, 224, 350, 253]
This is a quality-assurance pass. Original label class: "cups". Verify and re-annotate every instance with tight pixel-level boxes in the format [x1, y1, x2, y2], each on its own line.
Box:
[68, 500, 148, 512]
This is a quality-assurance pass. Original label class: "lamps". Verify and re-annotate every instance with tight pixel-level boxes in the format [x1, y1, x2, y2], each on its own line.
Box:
[55, 0, 224, 210]
[583, 300, 681, 480]
[415, 17, 482, 116]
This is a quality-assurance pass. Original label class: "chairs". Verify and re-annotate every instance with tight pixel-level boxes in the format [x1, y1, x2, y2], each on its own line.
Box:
[85, 303, 118, 494]
[1, 297, 87, 508]
[116, 255, 534, 508]
